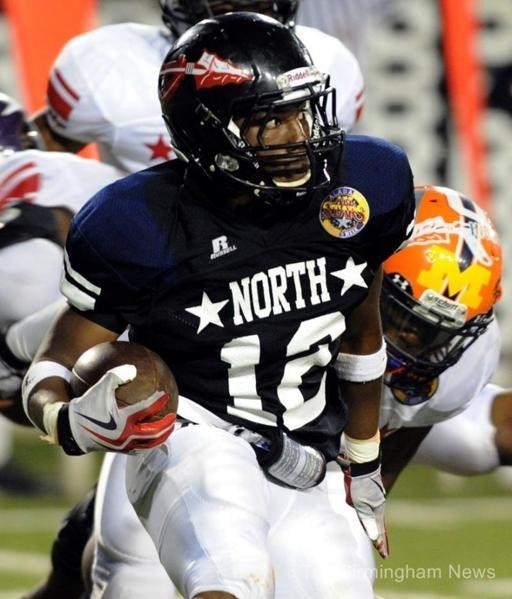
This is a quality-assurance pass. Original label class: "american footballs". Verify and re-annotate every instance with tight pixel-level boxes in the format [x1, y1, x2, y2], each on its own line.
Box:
[70, 341, 179, 424]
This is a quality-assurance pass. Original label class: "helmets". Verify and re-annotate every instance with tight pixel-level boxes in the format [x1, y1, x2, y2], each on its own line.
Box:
[158, 0, 346, 209]
[381, 184, 501, 391]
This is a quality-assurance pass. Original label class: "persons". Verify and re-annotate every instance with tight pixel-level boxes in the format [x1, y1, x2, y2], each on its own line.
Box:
[0, 0, 511, 599]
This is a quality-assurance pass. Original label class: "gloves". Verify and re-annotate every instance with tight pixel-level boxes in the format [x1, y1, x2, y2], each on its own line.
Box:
[57, 364, 176, 456]
[345, 448, 389, 560]
